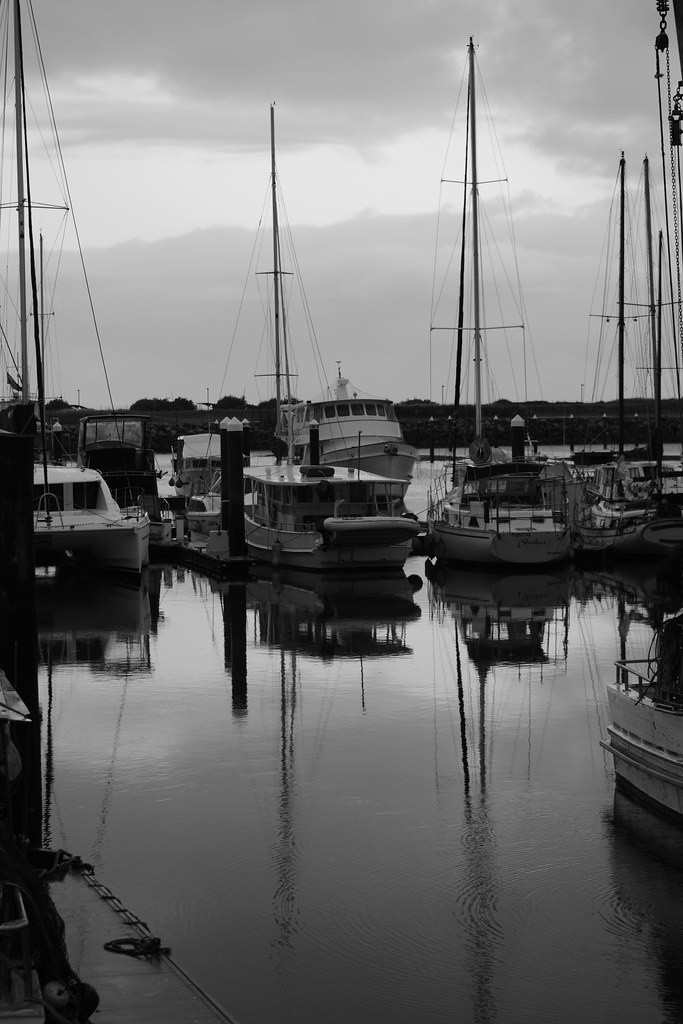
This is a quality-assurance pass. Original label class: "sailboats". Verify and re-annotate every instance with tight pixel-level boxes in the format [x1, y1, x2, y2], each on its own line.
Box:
[154, 97, 424, 572]
[562, 145, 682, 561]
[409, 28, 602, 566]
[216, 566, 430, 957]
[422, 554, 582, 828]
[1, 0, 154, 576]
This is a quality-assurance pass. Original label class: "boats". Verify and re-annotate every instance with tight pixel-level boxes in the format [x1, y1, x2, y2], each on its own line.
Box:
[277, 359, 421, 508]
